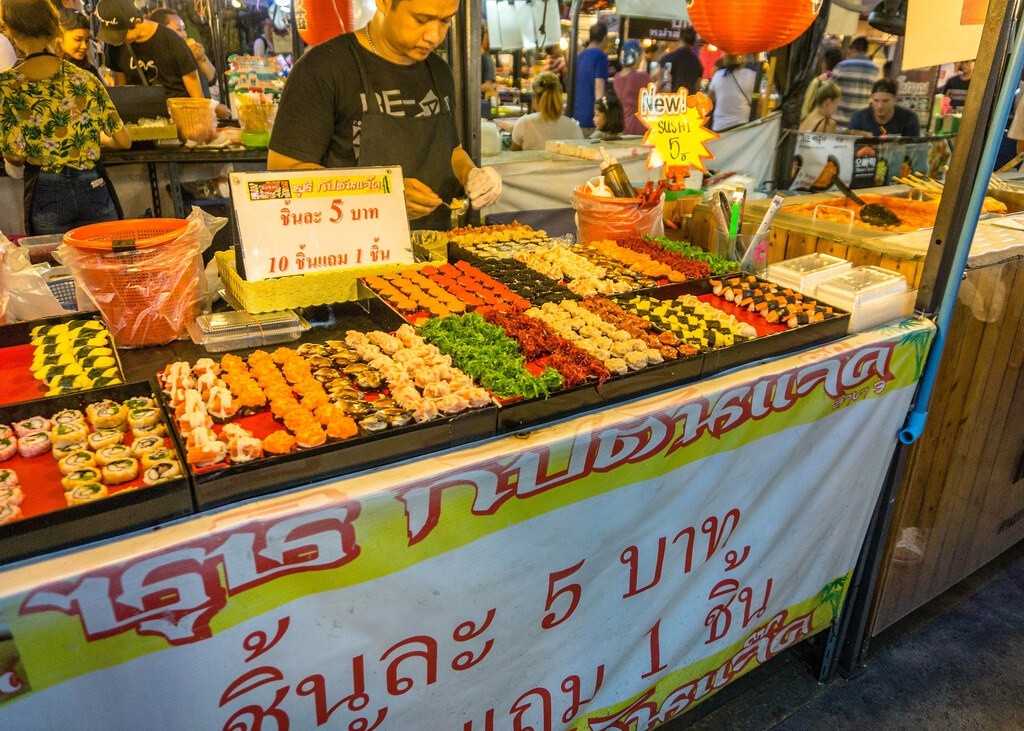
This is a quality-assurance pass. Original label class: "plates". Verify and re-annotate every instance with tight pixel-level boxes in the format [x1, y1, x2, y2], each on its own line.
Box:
[791, 155, 840, 191]
[875, 157, 889, 185]
[901, 155, 913, 175]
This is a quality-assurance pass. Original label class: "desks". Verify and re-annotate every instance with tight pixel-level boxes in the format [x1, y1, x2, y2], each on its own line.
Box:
[96, 128, 269, 219]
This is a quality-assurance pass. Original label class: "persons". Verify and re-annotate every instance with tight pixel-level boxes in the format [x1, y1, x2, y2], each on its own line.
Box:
[659, 27, 704, 96]
[574, 22, 664, 141]
[799, 36, 894, 133]
[521, 46, 567, 92]
[1008, 95, 1024, 155]
[849, 78, 921, 136]
[944, 60, 976, 108]
[96, 0, 203, 99]
[0, 1, 132, 236]
[708, 54, 762, 134]
[51, 0, 108, 86]
[481, 25, 495, 119]
[151, 8, 231, 120]
[254, 18, 273, 56]
[267, 0, 501, 232]
[511, 72, 584, 151]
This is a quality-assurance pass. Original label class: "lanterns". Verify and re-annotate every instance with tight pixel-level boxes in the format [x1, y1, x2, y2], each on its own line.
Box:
[686, 0, 824, 64]
[294, 0, 354, 46]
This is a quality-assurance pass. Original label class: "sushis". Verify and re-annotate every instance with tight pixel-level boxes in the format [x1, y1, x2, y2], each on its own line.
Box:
[0, 218, 847, 528]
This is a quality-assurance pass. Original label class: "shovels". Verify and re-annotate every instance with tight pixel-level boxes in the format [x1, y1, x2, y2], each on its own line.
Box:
[831, 174, 902, 227]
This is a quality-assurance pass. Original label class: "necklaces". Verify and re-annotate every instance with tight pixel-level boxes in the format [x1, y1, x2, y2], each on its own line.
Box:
[366, 21, 377, 53]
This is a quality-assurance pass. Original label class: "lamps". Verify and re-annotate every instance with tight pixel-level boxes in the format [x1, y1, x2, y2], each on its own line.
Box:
[560, 4, 572, 24]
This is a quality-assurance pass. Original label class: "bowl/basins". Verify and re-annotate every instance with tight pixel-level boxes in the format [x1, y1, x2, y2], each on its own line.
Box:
[241, 130, 272, 146]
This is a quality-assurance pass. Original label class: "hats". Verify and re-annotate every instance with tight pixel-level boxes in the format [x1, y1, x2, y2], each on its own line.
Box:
[619, 39, 641, 66]
[57, 8, 90, 29]
[96, 0, 137, 47]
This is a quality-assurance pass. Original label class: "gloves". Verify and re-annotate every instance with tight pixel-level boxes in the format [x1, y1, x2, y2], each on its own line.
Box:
[465, 166, 503, 211]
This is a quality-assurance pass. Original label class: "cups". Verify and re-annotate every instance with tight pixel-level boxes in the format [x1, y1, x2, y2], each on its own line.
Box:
[715, 222, 771, 277]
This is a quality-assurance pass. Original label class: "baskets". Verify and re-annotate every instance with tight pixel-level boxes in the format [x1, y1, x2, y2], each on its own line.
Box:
[46, 274, 77, 309]
[215, 243, 447, 314]
[236, 103, 276, 133]
[167, 97, 211, 141]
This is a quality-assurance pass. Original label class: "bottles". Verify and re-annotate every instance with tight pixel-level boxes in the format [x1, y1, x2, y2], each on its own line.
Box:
[599, 146, 636, 198]
